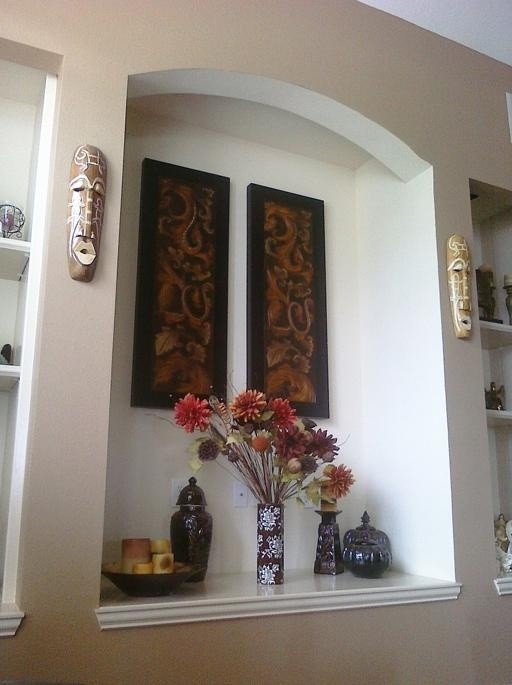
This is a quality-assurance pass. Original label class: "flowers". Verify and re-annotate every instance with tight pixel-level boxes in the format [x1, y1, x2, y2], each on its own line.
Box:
[141, 368, 357, 507]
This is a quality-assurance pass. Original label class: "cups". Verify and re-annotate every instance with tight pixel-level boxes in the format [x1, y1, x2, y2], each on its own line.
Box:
[121, 536, 173, 575]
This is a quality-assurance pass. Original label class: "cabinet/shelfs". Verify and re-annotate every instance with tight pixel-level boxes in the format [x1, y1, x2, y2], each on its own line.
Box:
[481, 318, 512, 428]
[1, 235, 32, 391]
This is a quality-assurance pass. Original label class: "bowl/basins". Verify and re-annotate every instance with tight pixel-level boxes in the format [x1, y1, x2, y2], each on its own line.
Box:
[96, 564, 192, 598]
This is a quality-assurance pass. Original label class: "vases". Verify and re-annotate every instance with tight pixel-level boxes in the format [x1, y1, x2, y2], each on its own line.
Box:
[256, 502, 284, 587]
